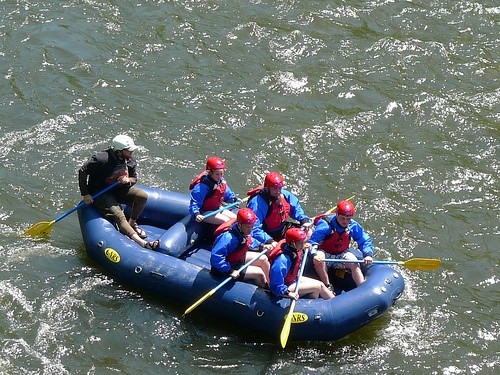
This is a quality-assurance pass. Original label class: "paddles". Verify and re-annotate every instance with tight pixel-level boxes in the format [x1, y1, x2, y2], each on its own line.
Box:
[202, 187, 265, 217]
[322, 259, 442, 270]
[182, 248, 268, 317]
[22, 180, 122, 236]
[300, 195, 356, 231]
[280, 247, 309, 349]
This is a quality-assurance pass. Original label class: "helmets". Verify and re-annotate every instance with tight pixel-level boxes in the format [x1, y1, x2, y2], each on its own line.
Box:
[236, 208, 256, 224]
[205, 157, 226, 171]
[264, 172, 283, 188]
[111, 134, 134, 151]
[336, 200, 355, 216]
[286, 228, 307, 245]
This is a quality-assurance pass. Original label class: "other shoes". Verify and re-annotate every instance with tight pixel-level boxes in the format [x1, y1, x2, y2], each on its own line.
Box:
[327, 283, 336, 295]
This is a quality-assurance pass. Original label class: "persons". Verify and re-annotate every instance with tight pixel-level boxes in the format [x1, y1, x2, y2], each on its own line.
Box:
[268, 228, 337, 300]
[246, 172, 313, 241]
[189, 157, 244, 225]
[308, 199, 373, 293]
[210, 207, 275, 289]
[79, 134, 160, 251]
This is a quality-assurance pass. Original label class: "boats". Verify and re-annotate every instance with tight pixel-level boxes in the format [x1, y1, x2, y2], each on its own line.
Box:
[76, 184, 405, 344]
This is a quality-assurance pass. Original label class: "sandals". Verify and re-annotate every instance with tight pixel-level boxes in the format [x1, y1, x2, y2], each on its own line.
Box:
[143, 239, 160, 250]
[131, 223, 146, 238]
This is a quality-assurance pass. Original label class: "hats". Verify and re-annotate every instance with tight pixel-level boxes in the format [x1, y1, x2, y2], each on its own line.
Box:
[126, 144, 136, 151]
